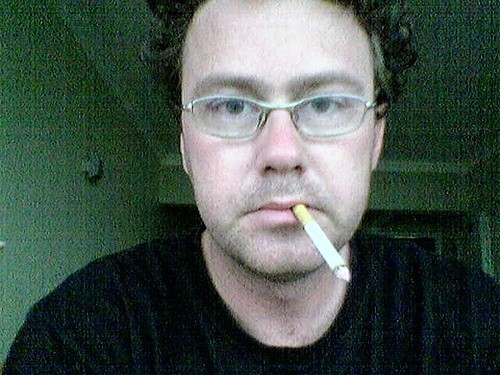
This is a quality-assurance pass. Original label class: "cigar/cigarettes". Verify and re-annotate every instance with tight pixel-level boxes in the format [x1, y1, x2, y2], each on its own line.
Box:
[292, 204, 350, 282]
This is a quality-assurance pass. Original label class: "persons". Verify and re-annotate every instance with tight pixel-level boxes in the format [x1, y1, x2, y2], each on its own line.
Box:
[0, 0, 500, 375]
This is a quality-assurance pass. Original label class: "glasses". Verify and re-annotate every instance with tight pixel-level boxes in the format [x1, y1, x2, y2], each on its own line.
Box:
[178, 94, 378, 138]
